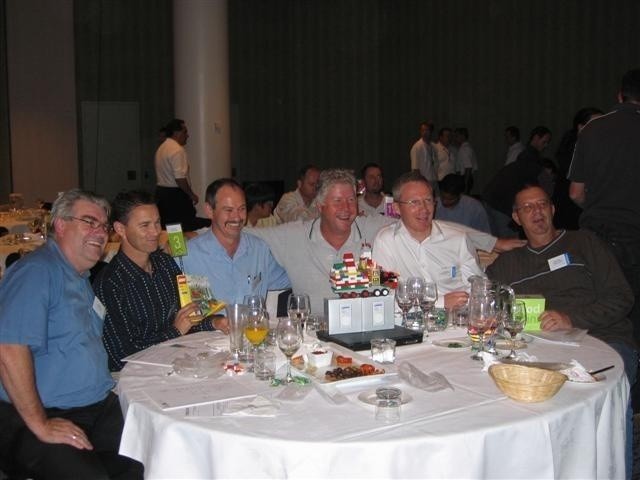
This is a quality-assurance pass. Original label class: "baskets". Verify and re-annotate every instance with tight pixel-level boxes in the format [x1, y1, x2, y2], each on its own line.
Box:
[489, 364, 567, 403]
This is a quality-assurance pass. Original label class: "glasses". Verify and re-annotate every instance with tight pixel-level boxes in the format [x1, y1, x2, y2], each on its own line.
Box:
[397, 198, 436, 207]
[63, 216, 113, 233]
[516, 199, 552, 212]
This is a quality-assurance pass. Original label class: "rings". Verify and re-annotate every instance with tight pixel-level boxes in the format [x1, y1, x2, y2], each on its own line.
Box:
[71, 435, 75, 439]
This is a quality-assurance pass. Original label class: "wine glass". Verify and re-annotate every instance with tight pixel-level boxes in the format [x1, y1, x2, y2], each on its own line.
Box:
[243, 293, 267, 309]
[485, 304, 505, 355]
[287, 294, 311, 346]
[502, 300, 526, 360]
[394, 283, 414, 328]
[244, 308, 270, 372]
[420, 283, 437, 335]
[470, 297, 496, 362]
[409, 277, 425, 329]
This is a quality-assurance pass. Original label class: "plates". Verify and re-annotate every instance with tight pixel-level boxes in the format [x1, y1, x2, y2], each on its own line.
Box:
[277, 340, 391, 392]
[432, 338, 472, 349]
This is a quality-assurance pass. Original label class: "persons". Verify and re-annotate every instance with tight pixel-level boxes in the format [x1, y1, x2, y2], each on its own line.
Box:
[566, 70, 638, 291]
[411, 122, 440, 196]
[435, 175, 487, 231]
[155, 119, 198, 230]
[244, 179, 283, 231]
[431, 129, 453, 179]
[276, 168, 321, 221]
[93, 190, 231, 371]
[166, 172, 527, 316]
[545, 107, 605, 227]
[371, 174, 489, 311]
[486, 183, 638, 475]
[450, 126, 477, 192]
[0, 188, 145, 480]
[491, 127, 549, 217]
[504, 127, 523, 164]
[172, 177, 293, 327]
[356, 164, 396, 216]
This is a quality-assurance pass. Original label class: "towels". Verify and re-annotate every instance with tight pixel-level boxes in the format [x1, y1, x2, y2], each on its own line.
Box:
[524, 328, 588, 347]
[219, 393, 287, 418]
[205, 335, 230, 352]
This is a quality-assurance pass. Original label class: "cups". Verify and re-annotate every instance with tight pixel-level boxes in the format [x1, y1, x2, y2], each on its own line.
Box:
[255, 352, 276, 381]
[424, 307, 451, 336]
[452, 303, 470, 329]
[277, 317, 303, 386]
[468, 273, 516, 332]
[374, 388, 400, 426]
[305, 313, 324, 338]
[226, 303, 248, 354]
